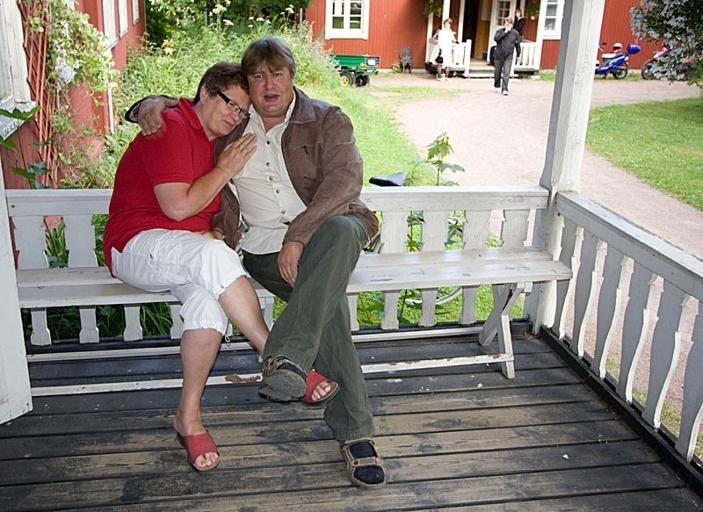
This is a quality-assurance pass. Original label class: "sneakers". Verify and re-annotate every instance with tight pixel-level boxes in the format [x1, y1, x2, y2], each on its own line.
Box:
[502, 89, 508, 95]
[495, 87, 500, 92]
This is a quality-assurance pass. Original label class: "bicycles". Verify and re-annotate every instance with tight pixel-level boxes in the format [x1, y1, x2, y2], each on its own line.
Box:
[363, 175, 464, 309]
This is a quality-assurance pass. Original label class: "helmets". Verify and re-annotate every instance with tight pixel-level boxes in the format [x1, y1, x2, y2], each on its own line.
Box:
[612, 43, 622, 53]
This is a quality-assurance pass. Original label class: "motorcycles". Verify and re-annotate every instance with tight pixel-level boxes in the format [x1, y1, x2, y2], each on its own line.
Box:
[587, 42, 700, 79]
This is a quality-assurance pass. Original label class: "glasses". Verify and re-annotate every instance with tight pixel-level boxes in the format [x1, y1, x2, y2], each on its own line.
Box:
[211, 85, 251, 121]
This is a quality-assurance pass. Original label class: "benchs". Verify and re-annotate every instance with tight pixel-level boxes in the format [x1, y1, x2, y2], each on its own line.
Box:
[15, 246, 573, 400]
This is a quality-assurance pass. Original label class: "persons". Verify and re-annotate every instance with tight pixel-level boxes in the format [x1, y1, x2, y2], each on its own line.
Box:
[490, 16, 521, 96]
[513, 10, 524, 41]
[100, 62, 341, 472]
[433, 17, 458, 83]
[122, 35, 387, 492]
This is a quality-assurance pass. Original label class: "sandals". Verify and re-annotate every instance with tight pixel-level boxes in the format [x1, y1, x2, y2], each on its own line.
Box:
[339, 438, 386, 489]
[258, 355, 307, 402]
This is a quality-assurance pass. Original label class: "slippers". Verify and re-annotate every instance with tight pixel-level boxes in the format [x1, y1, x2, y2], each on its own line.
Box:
[299, 369, 341, 407]
[177, 427, 221, 474]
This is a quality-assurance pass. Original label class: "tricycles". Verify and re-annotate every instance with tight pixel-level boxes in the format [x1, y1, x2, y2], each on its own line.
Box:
[328, 52, 380, 88]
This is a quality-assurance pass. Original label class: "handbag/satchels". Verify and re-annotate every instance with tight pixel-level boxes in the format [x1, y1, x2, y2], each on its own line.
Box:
[436, 56, 443, 63]
